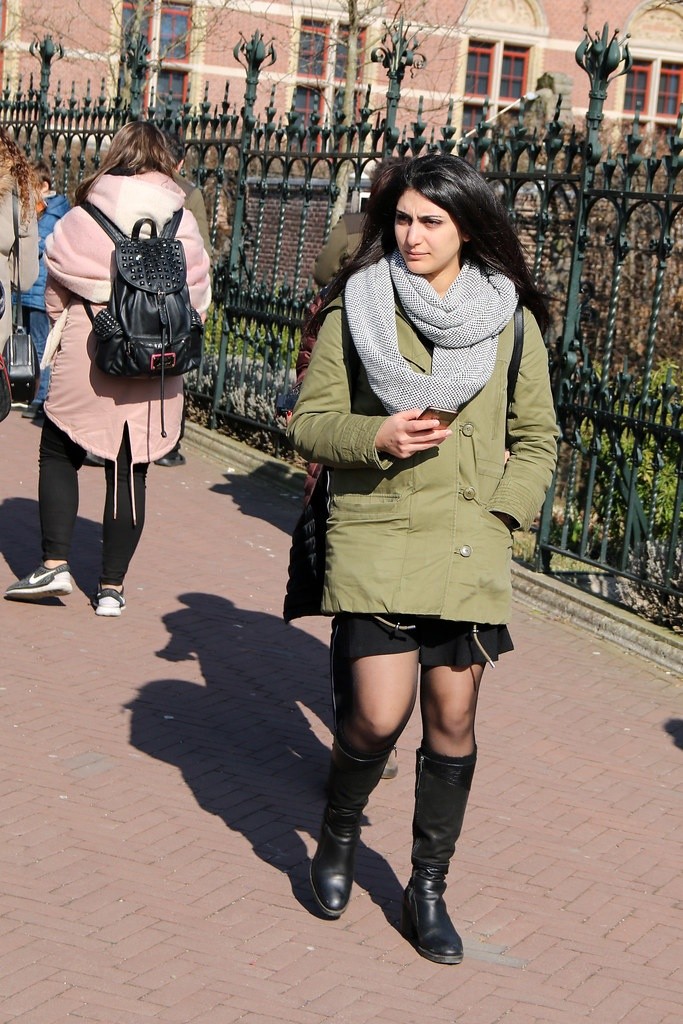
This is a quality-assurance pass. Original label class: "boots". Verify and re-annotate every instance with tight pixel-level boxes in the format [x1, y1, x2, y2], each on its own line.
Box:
[404, 744, 477, 963]
[309, 731, 397, 916]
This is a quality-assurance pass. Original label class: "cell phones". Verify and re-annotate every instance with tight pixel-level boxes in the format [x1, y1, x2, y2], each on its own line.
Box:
[416, 406, 458, 430]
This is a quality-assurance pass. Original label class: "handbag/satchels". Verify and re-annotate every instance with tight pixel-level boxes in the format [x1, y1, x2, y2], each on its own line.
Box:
[0, 354, 12, 422]
[2, 333, 35, 401]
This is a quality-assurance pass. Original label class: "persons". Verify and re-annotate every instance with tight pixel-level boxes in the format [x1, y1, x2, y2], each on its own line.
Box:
[0, 118, 416, 775]
[284, 152, 560, 964]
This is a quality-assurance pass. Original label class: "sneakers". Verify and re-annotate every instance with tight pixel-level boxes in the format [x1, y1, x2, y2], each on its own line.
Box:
[93, 581, 127, 616]
[3, 564, 73, 600]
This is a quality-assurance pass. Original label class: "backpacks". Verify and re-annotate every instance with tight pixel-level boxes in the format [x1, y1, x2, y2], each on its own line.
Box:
[77, 200, 204, 379]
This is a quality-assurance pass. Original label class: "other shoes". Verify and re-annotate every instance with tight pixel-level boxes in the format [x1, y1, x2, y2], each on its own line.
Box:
[22, 405, 39, 417]
[82, 452, 105, 467]
[154, 451, 185, 467]
